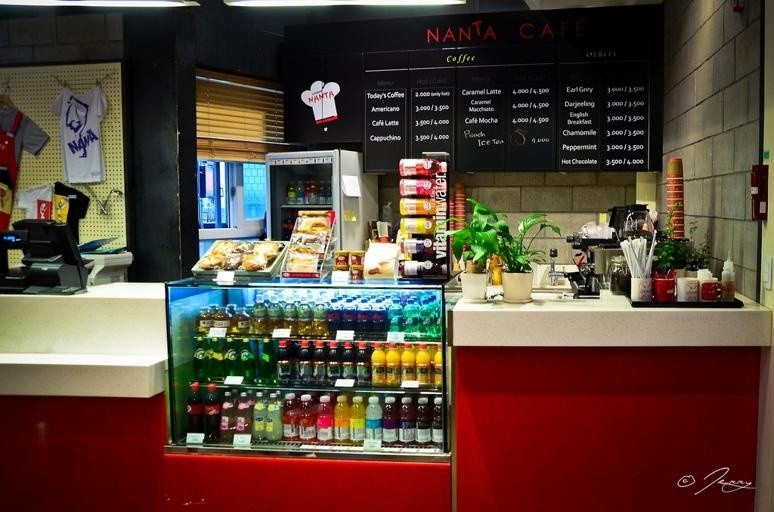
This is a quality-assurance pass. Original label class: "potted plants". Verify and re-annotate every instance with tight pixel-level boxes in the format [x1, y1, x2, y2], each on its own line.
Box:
[652, 205, 678, 302]
[453, 213, 500, 301]
[674, 214, 712, 279]
[433, 197, 561, 302]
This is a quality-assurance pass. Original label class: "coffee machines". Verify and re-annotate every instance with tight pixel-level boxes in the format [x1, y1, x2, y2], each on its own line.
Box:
[566, 236, 662, 299]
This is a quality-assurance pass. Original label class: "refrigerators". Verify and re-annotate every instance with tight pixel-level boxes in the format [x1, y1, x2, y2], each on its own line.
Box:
[263, 149, 377, 251]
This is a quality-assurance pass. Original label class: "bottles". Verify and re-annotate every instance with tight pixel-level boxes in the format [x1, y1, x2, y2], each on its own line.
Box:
[608, 257, 633, 294]
[282, 212, 298, 241]
[182, 292, 443, 445]
[368, 260, 394, 275]
[397, 159, 447, 278]
[491, 254, 503, 285]
[721, 251, 736, 301]
[286, 182, 331, 205]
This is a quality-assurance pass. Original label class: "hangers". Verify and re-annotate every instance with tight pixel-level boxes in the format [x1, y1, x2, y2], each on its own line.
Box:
[1, 74, 19, 110]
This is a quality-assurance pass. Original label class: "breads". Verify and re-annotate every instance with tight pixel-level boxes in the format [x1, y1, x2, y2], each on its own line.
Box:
[199, 241, 279, 271]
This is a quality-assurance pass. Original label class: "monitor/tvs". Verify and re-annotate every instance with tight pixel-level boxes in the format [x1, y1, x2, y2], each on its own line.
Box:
[13, 219, 87, 289]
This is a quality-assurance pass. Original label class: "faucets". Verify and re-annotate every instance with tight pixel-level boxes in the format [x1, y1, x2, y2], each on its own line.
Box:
[548, 258, 566, 286]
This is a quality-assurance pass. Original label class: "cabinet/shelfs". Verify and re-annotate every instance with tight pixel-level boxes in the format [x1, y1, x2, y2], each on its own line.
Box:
[164, 273, 450, 456]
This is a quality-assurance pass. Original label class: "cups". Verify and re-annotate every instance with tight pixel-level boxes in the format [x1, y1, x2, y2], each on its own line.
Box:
[676, 277, 697, 302]
[665, 158, 684, 239]
[447, 178, 465, 258]
[598, 212, 609, 225]
[379, 236, 390, 243]
[630, 278, 652, 302]
[653, 278, 677, 301]
[697, 278, 722, 301]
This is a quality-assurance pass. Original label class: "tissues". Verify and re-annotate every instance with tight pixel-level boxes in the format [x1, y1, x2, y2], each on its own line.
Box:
[376, 220, 394, 243]
